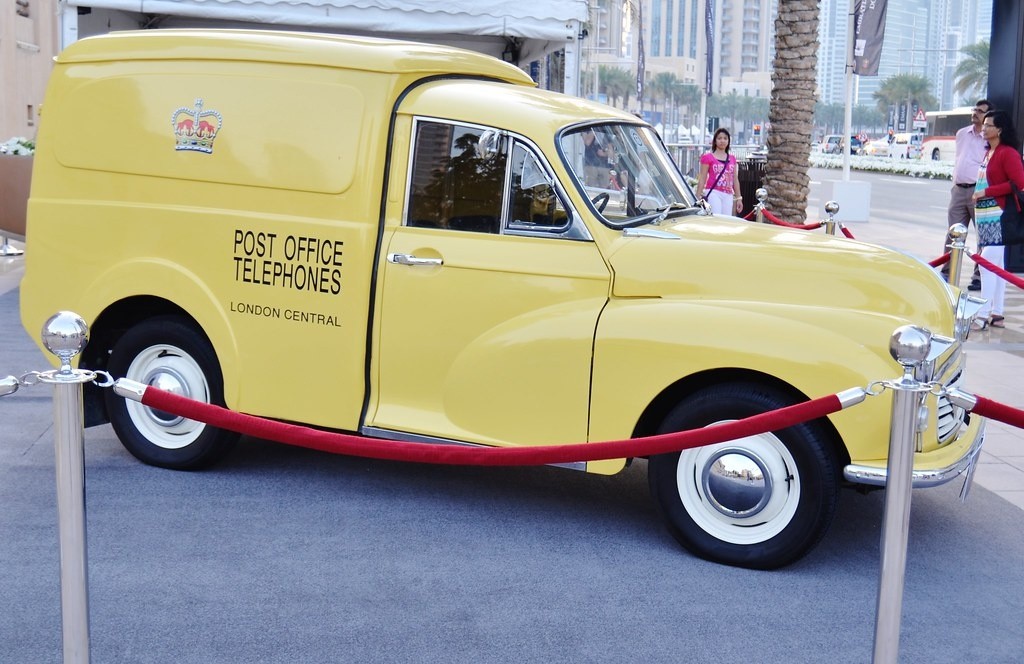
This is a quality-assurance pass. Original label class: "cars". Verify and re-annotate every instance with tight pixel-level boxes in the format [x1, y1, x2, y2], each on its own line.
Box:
[889, 133, 923, 159]
[864, 134, 889, 156]
[678, 134, 713, 145]
[812, 133, 863, 155]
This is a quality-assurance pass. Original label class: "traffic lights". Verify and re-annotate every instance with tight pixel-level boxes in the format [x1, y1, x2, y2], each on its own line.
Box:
[707, 117, 712, 133]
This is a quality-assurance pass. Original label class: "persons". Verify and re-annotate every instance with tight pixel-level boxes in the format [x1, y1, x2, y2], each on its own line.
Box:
[886, 130, 897, 158]
[970, 109, 1024, 332]
[938, 100, 995, 290]
[582, 113, 660, 197]
[696, 128, 743, 215]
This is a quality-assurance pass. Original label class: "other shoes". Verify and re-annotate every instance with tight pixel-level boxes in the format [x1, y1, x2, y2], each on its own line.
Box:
[941, 270, 949, 276]
[968, 279, 981, 290]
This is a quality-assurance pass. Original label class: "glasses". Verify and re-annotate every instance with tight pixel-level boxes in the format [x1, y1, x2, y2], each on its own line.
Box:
[982, 124, 995, 128]
[972, 108, 985, 113]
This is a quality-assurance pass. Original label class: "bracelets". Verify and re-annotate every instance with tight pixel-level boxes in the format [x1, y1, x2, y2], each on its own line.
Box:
[736, 197, 742, 200]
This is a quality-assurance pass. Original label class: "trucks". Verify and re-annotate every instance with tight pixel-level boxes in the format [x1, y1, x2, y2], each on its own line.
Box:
[20, 25, 992, 570]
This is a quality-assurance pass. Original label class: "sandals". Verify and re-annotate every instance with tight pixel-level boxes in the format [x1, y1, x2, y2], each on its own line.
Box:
[988, 317, 1005, 328]
[970, 319, 988, 331]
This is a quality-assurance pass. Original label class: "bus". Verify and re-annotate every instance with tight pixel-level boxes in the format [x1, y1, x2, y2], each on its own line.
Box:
[922, 111, 976, 164]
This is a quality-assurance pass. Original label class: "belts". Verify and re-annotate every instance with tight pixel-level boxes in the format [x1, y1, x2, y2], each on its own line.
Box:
[956, 183, 976, 188]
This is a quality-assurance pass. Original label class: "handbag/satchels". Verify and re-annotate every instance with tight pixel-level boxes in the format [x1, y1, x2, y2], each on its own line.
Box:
[1000, 181, 1024, 244]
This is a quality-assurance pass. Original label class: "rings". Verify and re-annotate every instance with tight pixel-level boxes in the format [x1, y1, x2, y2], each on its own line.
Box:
[973, 197, 975, 200]
